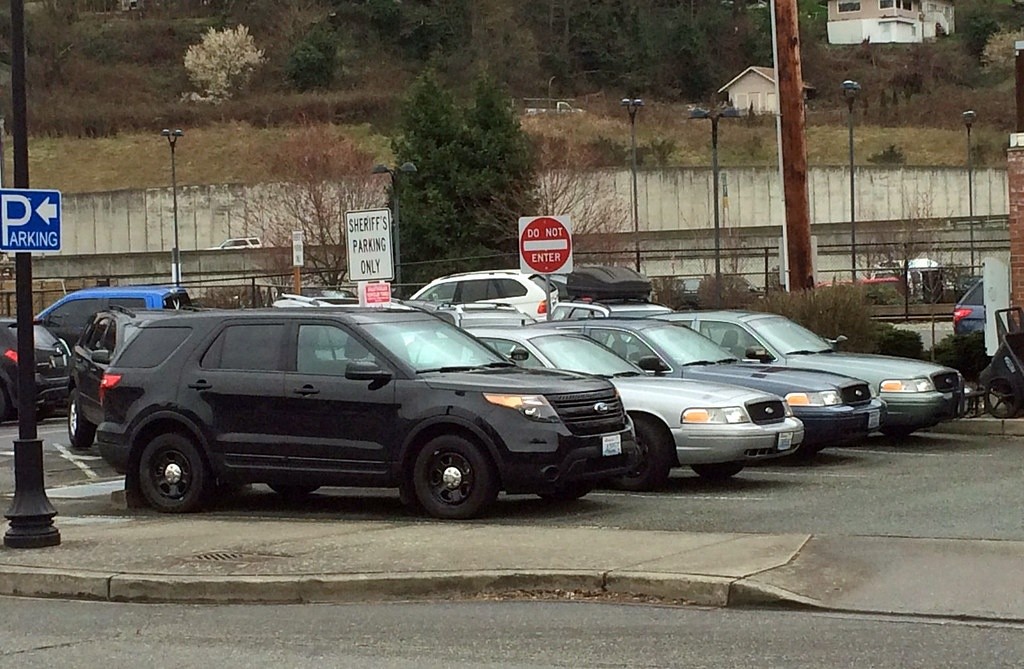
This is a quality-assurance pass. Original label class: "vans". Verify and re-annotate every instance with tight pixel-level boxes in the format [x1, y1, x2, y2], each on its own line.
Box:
[33, 287, 193, 348]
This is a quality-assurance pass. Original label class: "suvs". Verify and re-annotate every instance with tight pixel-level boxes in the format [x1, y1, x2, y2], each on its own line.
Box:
[94, 306, 646, 521]
[66, 304, 203, 451]
[208, 238, 263, 251]
[409, 269, 565, 328]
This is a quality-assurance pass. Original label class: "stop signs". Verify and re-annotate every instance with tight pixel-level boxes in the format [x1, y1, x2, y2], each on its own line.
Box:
[520, 216, 572, 273]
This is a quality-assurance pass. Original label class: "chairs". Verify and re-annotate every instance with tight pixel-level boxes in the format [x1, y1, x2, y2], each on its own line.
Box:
[345, 335, 368, 362]
[611, 340, 627, 359]
[721, 330, 738, 353]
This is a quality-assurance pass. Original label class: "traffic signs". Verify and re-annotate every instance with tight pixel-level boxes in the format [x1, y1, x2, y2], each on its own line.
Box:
[0, 189, 64, 253]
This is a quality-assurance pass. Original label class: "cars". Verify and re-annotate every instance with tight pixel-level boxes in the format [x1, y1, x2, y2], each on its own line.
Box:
[510, 316, 890, 467]
[270, 264, 760, 365]
[627, 308, 968, 449]
[0, 317, 74, 424]
[400, 328, 805, 492]
[815, 258, 987, 337]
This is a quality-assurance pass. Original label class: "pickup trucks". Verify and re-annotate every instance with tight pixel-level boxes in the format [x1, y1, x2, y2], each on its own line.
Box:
[524, 100, 585, 116]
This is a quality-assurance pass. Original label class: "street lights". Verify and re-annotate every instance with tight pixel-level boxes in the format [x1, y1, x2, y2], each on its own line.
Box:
[842, 80, 862, 283]
[161, 129, 185, 287]
[621, 98, 646, 273]
[963, 110, 978, 277]
[689, 107, 741, 281]
[371, 162, 419, 298]
